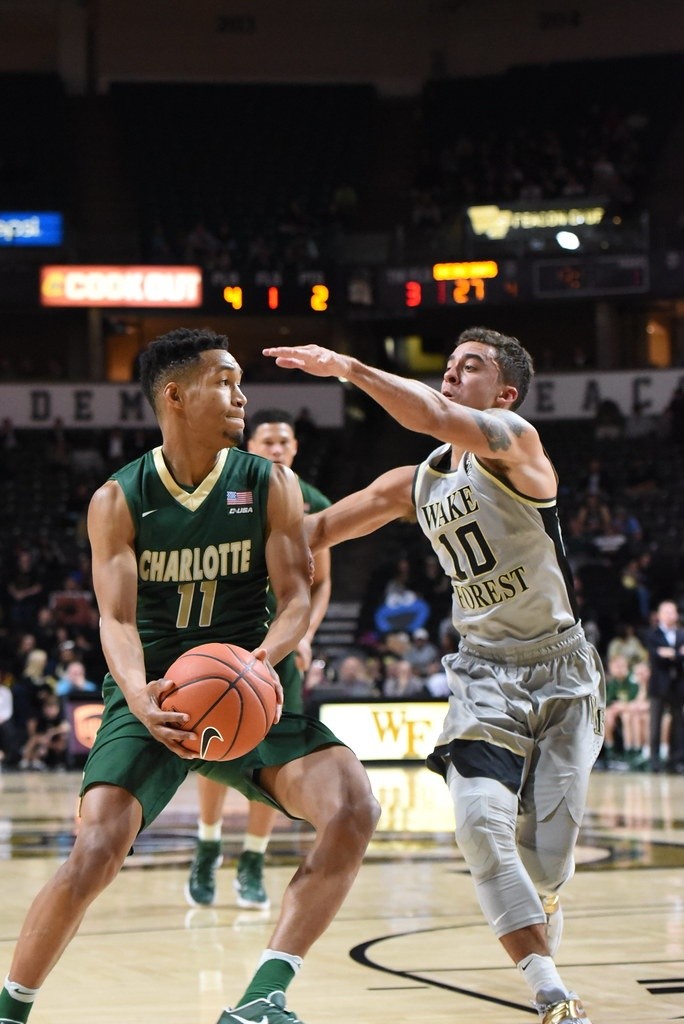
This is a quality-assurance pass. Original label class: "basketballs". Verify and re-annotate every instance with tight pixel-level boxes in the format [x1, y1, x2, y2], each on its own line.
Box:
[157, 642, 278, 763]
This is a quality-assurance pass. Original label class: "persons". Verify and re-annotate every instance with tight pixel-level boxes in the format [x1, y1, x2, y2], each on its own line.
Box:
[183, 407, 331, 910]
[262, 329, 608, 1024]
[0, 329, 381, 1024]
[0, 338, 684, 776]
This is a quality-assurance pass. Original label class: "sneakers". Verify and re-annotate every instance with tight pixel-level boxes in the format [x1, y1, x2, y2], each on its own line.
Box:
[233, 851, 270, 910]
[185, 838, 224, 908]
[217, 991, 306, 1024]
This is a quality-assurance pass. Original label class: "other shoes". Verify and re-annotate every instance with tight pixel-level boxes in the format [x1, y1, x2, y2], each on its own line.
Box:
[538, 895, 564, 957]
[530, 987, 593, 1024]
[604, 748, 684, 772]
